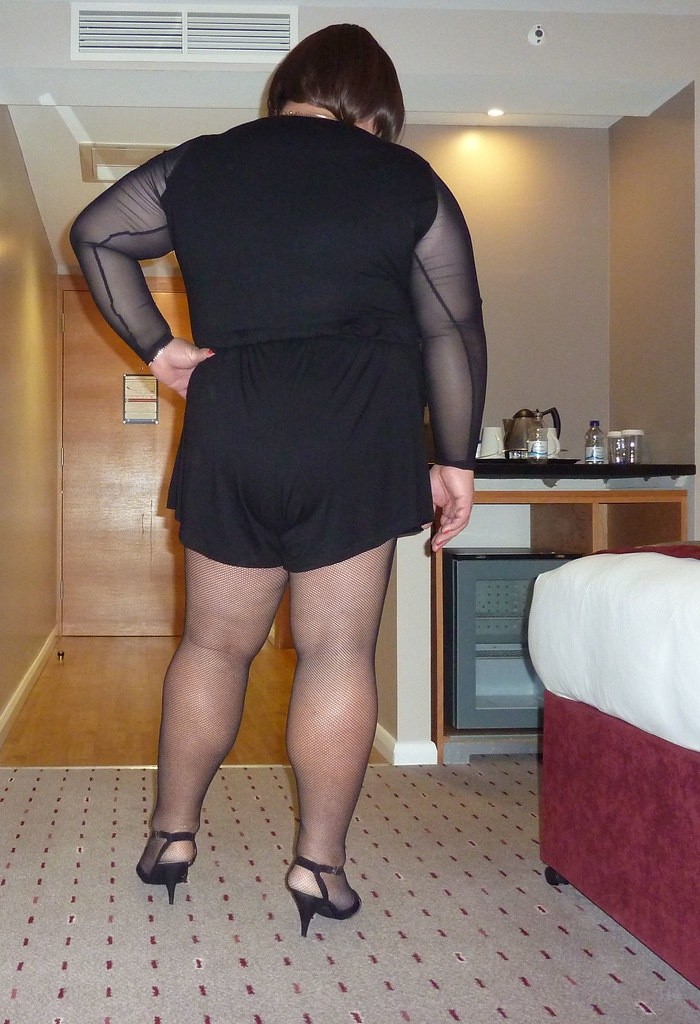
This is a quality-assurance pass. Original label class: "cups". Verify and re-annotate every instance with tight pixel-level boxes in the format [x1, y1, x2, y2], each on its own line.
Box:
[621, 429, 644, 464]
[606, 431, 624, 464]
[537, 428, 560, 459]
[480, 427, 506, 460]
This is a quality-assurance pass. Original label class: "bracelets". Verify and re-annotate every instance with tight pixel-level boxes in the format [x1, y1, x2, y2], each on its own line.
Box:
[148, 347, 165, 366]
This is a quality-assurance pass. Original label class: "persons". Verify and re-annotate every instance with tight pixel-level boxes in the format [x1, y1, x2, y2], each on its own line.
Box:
[70, 24, 488, 937]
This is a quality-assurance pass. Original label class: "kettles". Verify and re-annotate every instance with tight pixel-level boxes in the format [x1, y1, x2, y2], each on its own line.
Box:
[502, 407, 561, 460]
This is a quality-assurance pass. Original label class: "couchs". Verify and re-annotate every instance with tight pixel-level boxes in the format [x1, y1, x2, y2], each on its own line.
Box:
[525, 539, 700, 990]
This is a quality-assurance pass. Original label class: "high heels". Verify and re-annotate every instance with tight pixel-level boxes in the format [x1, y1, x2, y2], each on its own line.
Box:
[287, 856, 361, 938]
[138, 829, 197, 905]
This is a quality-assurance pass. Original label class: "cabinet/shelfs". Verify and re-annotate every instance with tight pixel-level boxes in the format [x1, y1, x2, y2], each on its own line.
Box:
[371, 462, 695, 767]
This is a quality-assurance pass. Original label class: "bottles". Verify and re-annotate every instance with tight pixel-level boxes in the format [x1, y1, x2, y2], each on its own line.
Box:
[585, 420, 604, 464]
[527, 426, 548, 464]
